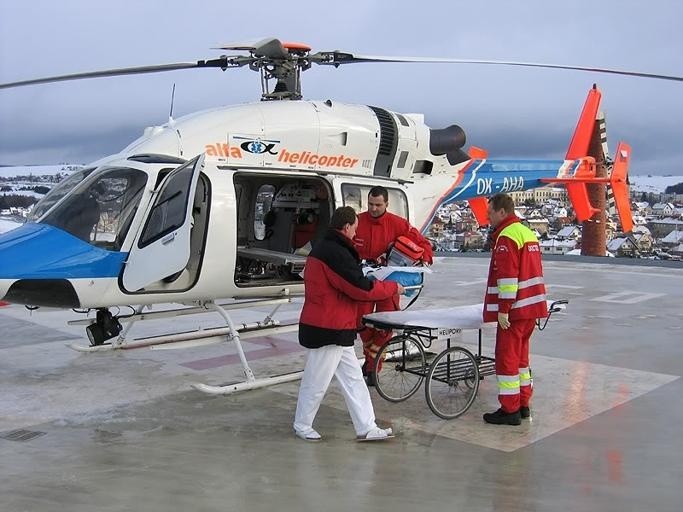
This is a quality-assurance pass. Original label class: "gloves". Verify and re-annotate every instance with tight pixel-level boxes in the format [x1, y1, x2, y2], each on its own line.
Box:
[497, 312, 511, 330]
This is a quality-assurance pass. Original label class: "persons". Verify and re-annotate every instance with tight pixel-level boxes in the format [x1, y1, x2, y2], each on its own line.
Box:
[349, 185, 433, 387]
[482, 192, 549, 427]
[290, 206, 408, 443]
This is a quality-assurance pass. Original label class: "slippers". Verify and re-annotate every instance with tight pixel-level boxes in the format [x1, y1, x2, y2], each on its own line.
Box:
[292, 424, 322, 442]
[356, 426, 396, 442]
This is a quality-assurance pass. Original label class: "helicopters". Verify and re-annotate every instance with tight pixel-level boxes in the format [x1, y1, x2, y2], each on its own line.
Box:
[0, 37, 683, 399]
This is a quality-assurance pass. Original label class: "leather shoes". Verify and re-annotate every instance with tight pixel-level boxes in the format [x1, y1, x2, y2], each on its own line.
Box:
[519, 407, 531, 418]
[362, 361, 369, 376]
[366, 372, 380, 386]
[483, 408, 521, 426]
